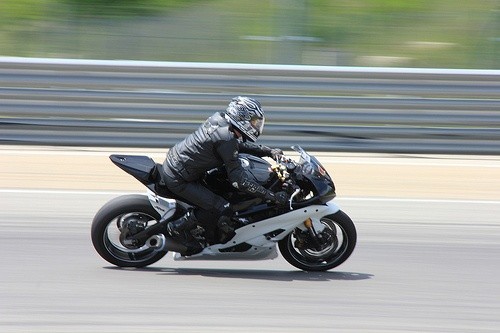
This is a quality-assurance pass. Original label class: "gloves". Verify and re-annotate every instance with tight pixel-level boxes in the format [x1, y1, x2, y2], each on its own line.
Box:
[270, 148, 283, 160]
[275, 191, 289, 206]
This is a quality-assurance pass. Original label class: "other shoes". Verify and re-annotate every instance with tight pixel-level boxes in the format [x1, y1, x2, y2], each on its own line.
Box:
[167, 217, 202, 248]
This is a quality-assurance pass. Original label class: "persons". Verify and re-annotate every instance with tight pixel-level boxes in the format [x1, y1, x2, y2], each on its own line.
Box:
[162, 95, 289, 244]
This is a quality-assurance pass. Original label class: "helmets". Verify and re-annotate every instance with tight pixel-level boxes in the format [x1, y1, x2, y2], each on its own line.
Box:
[224, 97, 264, 142]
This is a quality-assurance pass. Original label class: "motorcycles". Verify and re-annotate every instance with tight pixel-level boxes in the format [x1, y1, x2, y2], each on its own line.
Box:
[91, 145, 357, 272]
